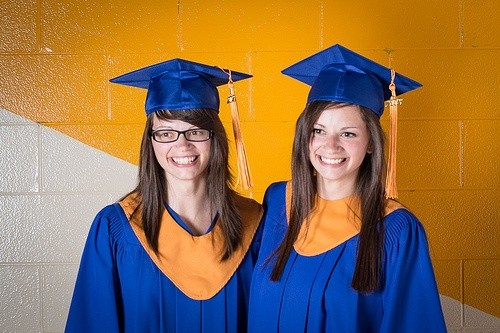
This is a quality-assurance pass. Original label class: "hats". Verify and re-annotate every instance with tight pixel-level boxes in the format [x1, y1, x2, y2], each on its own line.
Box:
[108, 57, 256, 192]
[280, 43, 424, 200]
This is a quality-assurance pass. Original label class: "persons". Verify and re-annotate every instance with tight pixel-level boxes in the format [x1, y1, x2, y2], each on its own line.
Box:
[64, 59, 266, 333]
[246, 44, 447, 333]
